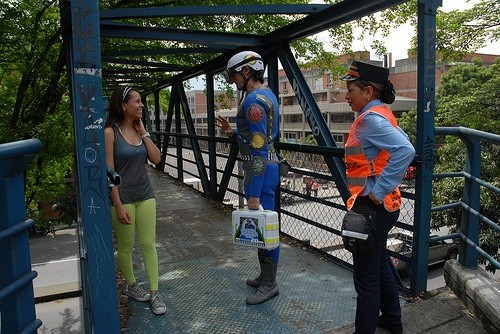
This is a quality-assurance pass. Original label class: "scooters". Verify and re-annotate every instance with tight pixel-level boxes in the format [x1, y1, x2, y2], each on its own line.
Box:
[281, 193, 296, 205]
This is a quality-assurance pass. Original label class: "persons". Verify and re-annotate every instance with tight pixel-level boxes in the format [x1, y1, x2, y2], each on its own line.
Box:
[213, 51, 280, 305]
[105, 85, 167, 315]
[339, 60, 415, 334]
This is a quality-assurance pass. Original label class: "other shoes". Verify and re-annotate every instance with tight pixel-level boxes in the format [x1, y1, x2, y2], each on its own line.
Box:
[150, 295, 166, 315]
[123, 280, 150, 301]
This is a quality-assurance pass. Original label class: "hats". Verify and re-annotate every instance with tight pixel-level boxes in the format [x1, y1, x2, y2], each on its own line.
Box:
[338, 60, 390, 85]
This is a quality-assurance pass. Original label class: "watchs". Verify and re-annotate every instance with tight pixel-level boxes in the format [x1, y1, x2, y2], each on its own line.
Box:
[141, 132, 150, 139]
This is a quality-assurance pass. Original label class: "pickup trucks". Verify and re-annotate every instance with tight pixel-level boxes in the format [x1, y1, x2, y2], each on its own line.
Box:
[385, 232, 457, 279]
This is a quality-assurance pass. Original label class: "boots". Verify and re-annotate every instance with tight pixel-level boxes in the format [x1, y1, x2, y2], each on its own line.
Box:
[247, 249, 262, 287]
[246, 256, 279, 305]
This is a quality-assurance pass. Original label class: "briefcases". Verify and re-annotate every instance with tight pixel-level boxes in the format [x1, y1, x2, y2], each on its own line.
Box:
[231, 202, 281, 251]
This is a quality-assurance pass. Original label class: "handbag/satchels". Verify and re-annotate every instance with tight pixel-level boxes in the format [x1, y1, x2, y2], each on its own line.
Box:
[341, 205, 381, 254]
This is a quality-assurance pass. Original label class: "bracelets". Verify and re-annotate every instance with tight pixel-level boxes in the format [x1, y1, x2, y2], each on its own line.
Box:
[114, 201, 121, 206]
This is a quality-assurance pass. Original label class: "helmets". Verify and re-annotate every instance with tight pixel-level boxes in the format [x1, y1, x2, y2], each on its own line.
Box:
[226, 49, 265, 77]
